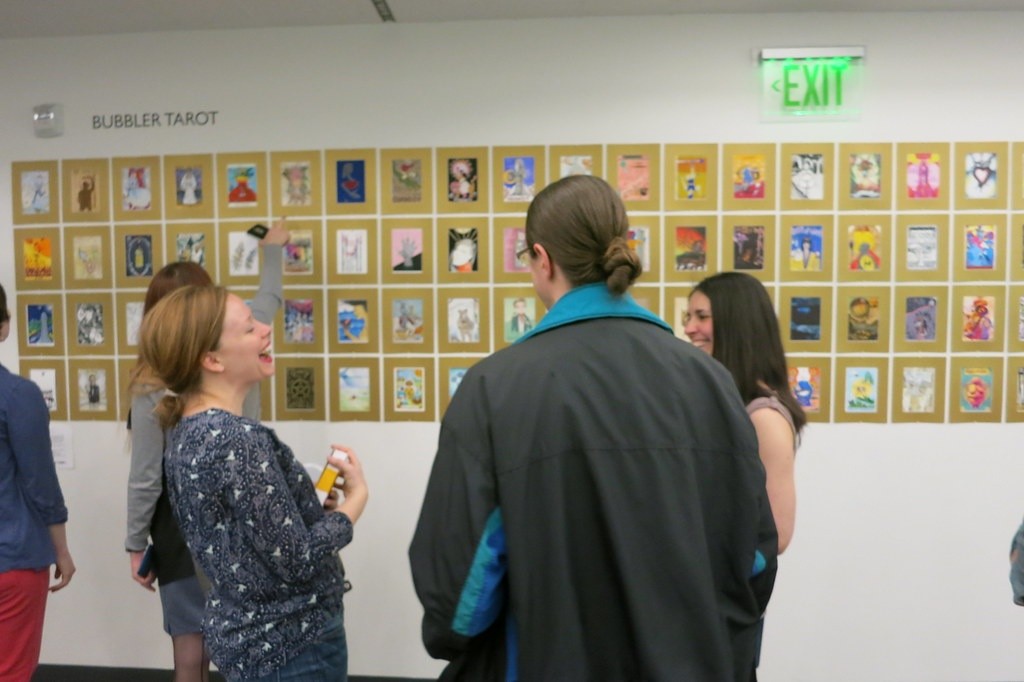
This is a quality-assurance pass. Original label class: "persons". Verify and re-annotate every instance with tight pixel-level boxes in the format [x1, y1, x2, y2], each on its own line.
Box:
[139, 282, 370, 682]
[0, 285, 76, 682]
[684, 272, 807, 552]
[407, 175, 778, 682]
[124, 219, 294, 682]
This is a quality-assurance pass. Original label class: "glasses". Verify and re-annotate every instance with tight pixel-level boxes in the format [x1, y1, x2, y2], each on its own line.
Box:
[516, 244, 540, 264]
